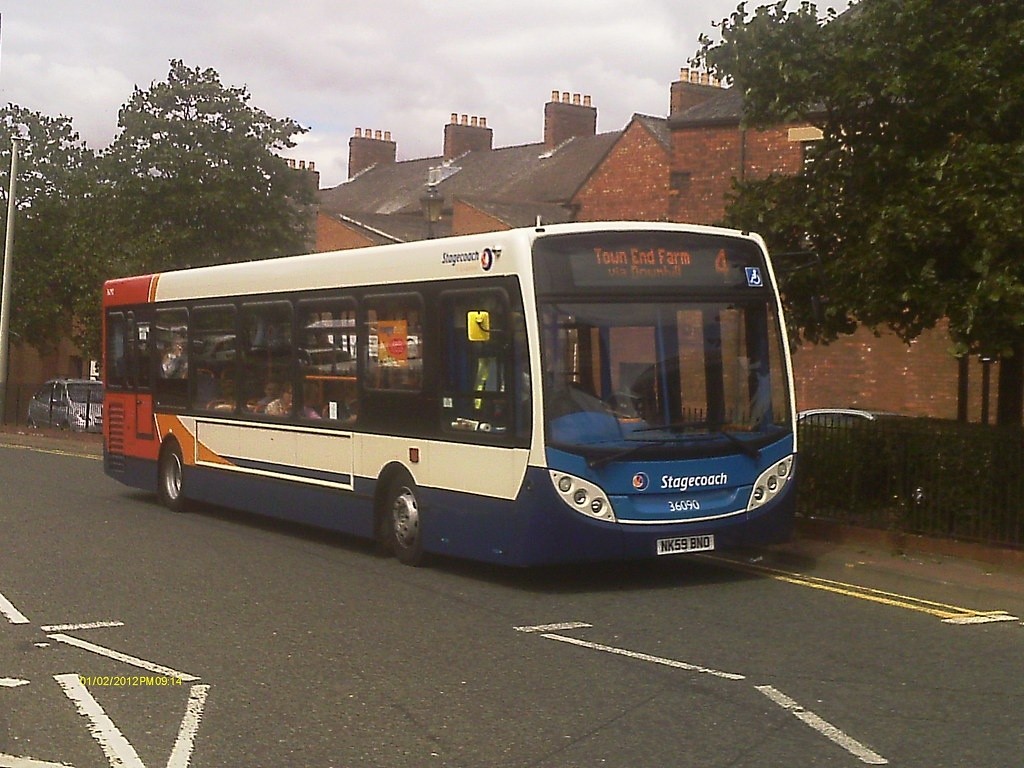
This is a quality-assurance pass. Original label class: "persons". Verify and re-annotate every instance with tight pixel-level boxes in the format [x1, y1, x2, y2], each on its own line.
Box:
[162, 328, 224, 377]
[255, 379, 318, 417]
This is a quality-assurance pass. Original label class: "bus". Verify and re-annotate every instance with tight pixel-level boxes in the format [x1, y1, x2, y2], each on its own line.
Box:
[102, 220, 797, 569]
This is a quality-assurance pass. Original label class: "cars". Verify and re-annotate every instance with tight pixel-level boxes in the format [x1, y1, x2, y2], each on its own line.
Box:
[299, 347, 357, 376]
[796, 407, 898, 425]
[27, 378, 103, 432]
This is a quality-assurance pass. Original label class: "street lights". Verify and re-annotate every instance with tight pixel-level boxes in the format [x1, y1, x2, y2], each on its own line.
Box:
[419, 179, 444, 239]
[0, 135, 31, 420]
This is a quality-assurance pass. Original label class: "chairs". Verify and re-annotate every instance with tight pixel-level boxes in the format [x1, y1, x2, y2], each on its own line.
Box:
[248, 400, 268, 414]
[221, 368, 254, 379]
[207, 400, 237, 412]
[181, 367, 215, 378]
